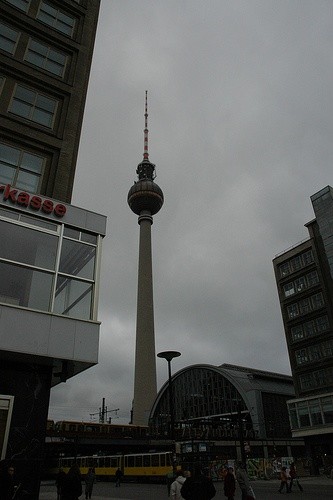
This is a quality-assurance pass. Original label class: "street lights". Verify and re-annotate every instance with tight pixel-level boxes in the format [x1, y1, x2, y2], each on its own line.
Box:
[157, 350, 182, 480]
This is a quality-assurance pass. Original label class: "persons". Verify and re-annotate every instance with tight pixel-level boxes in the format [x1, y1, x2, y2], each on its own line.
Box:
[168, 459, 216, 500]
[114, 468, 121, 487]
[57, 464, 82, 500]
[3, 466, 16, 480]
[85, 465, 95, 500]
[221, 467, 236, 500]
[290, 462, 304, 490]
[235, 461, 257, 500]
[278, 466, 290, 493]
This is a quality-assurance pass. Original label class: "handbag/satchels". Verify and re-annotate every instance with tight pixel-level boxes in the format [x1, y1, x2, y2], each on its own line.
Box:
[245, 486, 256, 499]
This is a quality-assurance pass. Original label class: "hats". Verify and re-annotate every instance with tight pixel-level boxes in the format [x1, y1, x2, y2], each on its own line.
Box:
[226, 465, 234, 471]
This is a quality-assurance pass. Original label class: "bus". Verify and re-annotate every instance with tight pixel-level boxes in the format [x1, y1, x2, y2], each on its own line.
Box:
[59, 453, 181, 483]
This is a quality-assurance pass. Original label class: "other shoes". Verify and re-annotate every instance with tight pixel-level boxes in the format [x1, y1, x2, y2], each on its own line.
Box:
[278, 490, 291, 494]
